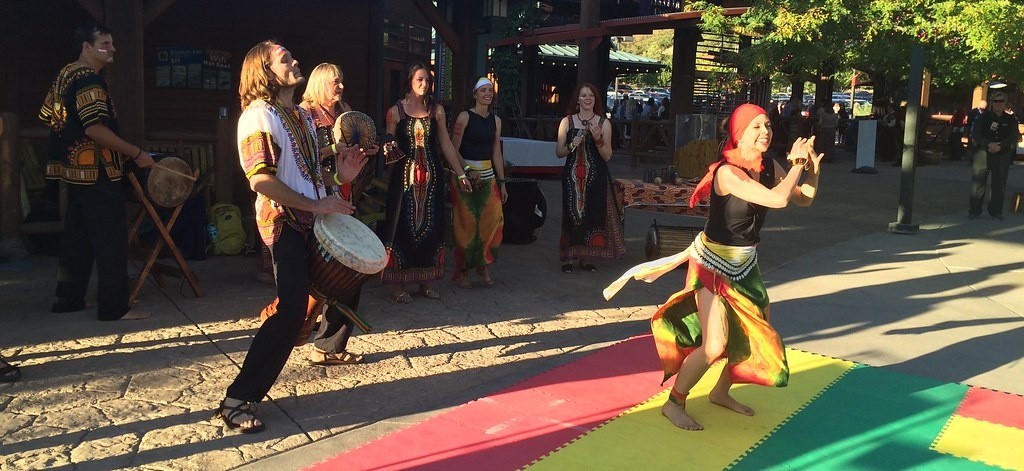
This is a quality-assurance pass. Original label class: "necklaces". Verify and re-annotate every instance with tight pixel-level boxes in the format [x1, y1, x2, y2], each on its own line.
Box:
[576, 112, 596, 126]
[317, 102, 338, 126]
[245, 96, 324, 188]
[474, 106, 487, 117]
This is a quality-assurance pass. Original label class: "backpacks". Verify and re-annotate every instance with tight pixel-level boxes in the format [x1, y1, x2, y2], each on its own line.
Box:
[206, 204, 247, 256]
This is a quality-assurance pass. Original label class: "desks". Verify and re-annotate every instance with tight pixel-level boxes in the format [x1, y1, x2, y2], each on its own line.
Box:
[613, 178, 710, 234]
[500, 137, 567, 178]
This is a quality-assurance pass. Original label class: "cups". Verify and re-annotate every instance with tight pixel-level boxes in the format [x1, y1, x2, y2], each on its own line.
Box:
[219, 107, 229, 120]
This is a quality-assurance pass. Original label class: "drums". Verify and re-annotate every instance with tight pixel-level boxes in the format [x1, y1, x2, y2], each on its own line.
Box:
[259, 212, 388, 347]
[642, 222, 704, 264]
[121, 149, 194, 209]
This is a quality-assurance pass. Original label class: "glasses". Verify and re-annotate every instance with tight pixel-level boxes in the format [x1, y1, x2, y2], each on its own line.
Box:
[992, 100, 1004, 104]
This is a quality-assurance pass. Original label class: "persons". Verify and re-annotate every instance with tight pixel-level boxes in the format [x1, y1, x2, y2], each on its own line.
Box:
[609, 95, 848, 149]
[379, 62, 472, 304]
[36, 21, 156, 320]
[662, 103, 826, 431]
[950, 100, 1018, 166]
[213, 39, 387, 434]
[967, 91, 1018, 220]
[556, 83, 614, 273]
[446, 77, 508, 290]
[0, 358, 22, 383]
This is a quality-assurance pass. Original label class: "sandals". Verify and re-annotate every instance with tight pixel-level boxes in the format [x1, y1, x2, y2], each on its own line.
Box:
[305, 345, 366, 365]
[0, 359, 21, 381]
[218, 395, 266, 434]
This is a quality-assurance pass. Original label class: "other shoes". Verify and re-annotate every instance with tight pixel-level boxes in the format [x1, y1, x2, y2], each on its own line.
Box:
[892, 162, 901, 167]
[969, 212, 977, 220]
[991, 214, 1004, 221]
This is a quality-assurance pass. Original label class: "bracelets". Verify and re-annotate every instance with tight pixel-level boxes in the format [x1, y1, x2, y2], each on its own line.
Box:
[333, 173, 343, 186]
[457, 165, 470, 179]
[593, 136, 604, 146]
[566, 142, 573, 152]
[785, 157, 811, 171]
[499, 179, 507, 182]
[130, 146, 141, 160]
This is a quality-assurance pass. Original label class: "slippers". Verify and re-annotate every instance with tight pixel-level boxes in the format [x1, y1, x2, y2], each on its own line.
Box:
[394, 289, 413, 304]
[583, 260, 598, 272]
[459, 272, 472, 291]
[562, 262, 572, 274]
[478, 267, 495, 287]
[425, 286, 440, 300]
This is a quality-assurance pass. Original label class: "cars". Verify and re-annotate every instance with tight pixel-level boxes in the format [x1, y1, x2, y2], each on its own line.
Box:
[608, 83, 670, 101]
[693, 92, 872, 111]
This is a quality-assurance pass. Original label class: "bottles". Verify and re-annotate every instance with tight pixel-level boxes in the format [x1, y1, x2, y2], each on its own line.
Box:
[208, 223, 218, 239]
[647, 222, 660, 260]
